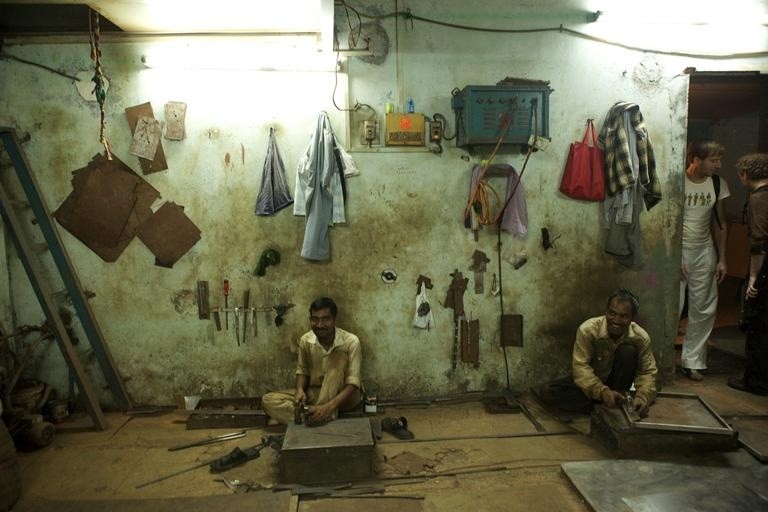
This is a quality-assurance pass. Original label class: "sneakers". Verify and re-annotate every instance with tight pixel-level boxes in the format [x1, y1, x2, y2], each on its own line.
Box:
[680, 365, 704, 381]
[727, 376, 768, 397]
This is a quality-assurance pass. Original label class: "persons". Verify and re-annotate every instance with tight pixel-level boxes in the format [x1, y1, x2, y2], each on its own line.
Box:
[676, 141, 731, 383]
[536, 288, 659, 421]
[261, 298, 365, 427]
[726, 152, 768, 397]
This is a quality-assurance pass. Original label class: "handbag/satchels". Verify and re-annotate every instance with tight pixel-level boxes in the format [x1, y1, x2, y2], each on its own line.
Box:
[559, 119, 606, 203]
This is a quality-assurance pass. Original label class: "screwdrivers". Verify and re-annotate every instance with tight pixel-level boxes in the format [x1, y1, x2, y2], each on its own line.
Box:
[223, 279, 230, 332]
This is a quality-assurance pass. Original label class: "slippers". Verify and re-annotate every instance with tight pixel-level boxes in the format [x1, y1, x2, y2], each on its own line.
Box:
[381, 415, 415, 440]
[209, 446, 261, 472]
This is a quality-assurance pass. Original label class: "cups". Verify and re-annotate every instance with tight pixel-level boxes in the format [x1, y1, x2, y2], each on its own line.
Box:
[385, 103, 395, 113]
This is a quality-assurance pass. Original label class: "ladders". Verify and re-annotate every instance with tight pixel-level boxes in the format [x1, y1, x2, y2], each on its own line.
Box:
[0, 125, 132, 431]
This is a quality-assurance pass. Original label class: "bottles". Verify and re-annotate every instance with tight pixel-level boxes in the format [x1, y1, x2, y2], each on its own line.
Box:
[407, 95, 414, 113]
